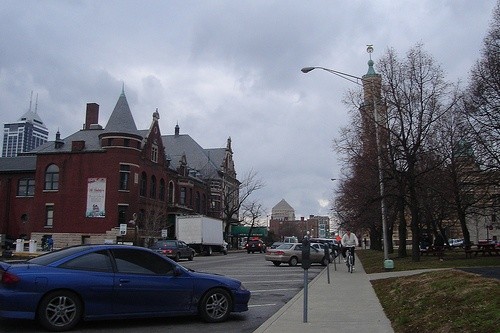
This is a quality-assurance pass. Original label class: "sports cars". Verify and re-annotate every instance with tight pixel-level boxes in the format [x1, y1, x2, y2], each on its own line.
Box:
[0, 243, 250, 333]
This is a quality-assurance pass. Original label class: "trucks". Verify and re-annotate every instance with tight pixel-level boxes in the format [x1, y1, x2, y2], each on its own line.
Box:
[175, 215, 227, 256]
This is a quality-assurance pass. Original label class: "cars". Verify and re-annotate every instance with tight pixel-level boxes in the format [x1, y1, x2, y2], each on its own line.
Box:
[270, 237, 342, 259]
[245, 239, 267, 254]
[264, 243, 333, 267]
[147, 240, 196, 262]
[444, 239, 464, 248]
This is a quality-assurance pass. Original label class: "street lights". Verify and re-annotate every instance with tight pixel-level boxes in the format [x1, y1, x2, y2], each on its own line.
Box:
[300, 66, 388, 262]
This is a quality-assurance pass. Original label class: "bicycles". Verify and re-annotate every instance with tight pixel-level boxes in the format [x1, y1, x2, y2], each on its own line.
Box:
[343, 245, 355, 273]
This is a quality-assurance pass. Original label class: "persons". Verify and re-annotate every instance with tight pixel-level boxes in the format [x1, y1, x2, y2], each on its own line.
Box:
[41, 236, 53, 251]
[340, 228, 359, 269]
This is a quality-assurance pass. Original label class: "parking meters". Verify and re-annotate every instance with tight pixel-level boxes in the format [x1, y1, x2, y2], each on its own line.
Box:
[301, 236, 311, 323]
[323, 243, 330, 285]
[332, 243, 337, 270]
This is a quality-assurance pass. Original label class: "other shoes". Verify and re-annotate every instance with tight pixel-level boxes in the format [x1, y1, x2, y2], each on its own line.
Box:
[352, 265, 355, 270]
[343, 256, 347, 262]
